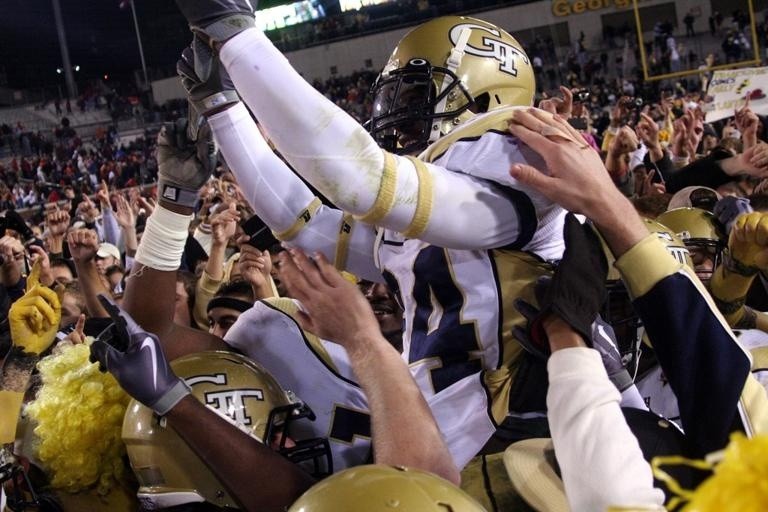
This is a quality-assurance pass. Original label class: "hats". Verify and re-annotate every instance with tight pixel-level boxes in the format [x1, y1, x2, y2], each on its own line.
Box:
[667, 186, 724, 212]
[504, 406, 685, 512]
[96, 242, 120, 260]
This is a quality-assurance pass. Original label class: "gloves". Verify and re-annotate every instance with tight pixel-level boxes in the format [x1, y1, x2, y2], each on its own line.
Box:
[0, 254, 65, 444]
[90, 293, 193, 416]
[177, 41, 240, 141]
[710, 211, 768, 329]
[512, 211, 634, 392]
[157, 116, 218, 208]
[179, 0, 258, 83]
[714, 196, 753, 235]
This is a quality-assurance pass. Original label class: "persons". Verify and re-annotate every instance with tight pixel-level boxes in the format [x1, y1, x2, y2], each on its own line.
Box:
[0, 0, 768, 511]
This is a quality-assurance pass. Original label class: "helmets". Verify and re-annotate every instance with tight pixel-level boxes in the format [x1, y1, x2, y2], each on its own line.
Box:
[363, 16, 536, 156]
[287, 464, 488, 512]
[122, 350, 333, 512]
[591, 218, 694, 383]
[654, 208, 729, 280]
